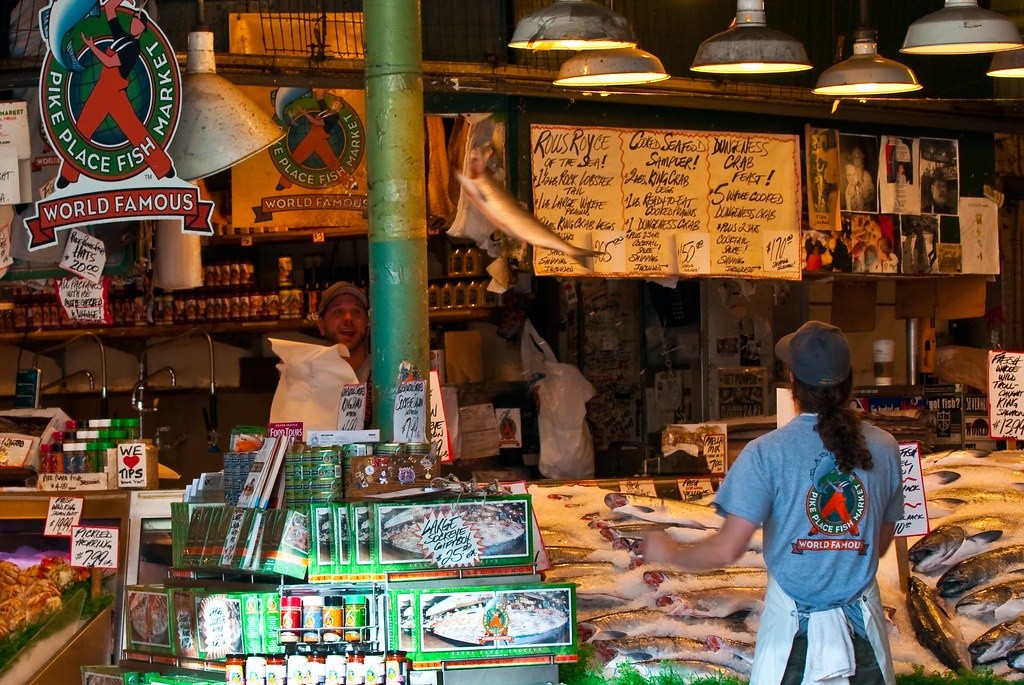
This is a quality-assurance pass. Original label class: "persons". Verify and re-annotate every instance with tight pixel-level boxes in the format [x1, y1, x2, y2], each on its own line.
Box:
[645, 321, 903, 685]
[318, 282, 373, 431]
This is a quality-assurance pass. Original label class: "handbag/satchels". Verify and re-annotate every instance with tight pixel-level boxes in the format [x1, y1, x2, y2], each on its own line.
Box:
[522, 318, 597, 481]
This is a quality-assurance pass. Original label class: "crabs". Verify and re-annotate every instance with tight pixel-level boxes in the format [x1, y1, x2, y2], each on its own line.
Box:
[0, 558, 107, 639]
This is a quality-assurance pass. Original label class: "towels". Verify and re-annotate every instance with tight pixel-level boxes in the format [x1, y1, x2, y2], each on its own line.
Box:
[800, 606, 856, 685]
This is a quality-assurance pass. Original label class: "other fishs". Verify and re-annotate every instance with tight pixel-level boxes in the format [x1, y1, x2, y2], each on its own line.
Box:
[454, 149, 607, 271]
[537, 447, 1024, 682]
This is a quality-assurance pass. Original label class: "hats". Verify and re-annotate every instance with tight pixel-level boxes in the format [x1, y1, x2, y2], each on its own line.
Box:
[775, 320, 851, 387]
[318, 280, 368, 314]
